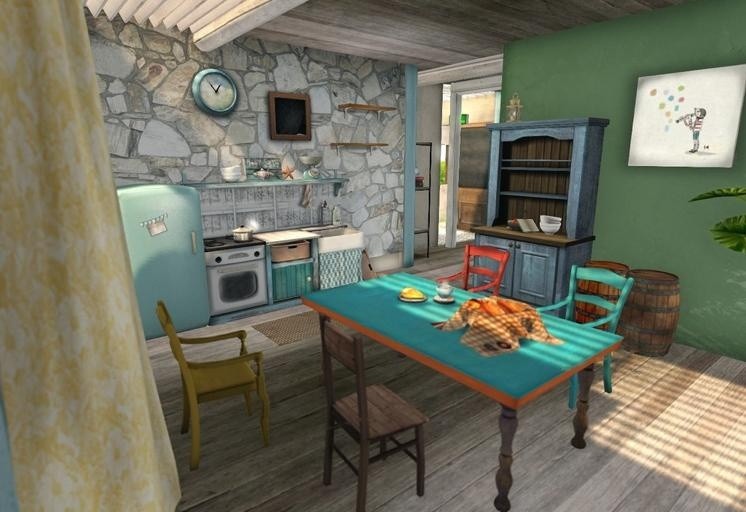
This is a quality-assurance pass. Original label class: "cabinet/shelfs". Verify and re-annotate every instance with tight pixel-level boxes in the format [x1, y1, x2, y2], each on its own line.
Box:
[179, 177, 345, 198]
[414, 142, 433, 257]
[202, 245, 267, 318]
[267, 240, 319, 306]
[471, 117, 610, 318]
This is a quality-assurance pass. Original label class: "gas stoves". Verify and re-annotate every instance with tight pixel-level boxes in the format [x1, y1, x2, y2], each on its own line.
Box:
[203, 234, 266, 268]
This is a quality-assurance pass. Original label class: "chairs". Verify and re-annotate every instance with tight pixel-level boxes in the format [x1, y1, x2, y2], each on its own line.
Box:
[156, 300, 272, 471]
[436, 243, 510, 296]
[318, 314, 430, 512]
[535, 265, 634, 409]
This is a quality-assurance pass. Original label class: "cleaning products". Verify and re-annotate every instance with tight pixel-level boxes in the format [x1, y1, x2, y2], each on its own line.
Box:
[332, 203, 342, 226]
[321, 200, 331, 225]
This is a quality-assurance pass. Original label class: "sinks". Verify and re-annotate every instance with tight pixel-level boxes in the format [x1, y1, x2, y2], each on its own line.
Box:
[308, 224, 359, 238]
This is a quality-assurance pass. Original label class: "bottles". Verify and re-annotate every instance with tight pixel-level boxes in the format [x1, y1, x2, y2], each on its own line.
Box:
[460, 114, 467, 125]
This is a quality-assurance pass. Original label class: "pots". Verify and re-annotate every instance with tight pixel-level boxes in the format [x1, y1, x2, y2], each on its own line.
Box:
[231, 225, 254, 242]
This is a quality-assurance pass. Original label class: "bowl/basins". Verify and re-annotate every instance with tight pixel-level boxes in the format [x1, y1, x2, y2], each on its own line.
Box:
[298, 155, 323, 165]
[219, 165, 242, 182]
[507, 219, 521, 228]
[540, 215, 562, 236]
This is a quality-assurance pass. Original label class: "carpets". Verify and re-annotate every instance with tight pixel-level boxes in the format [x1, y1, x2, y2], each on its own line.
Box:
[253, 310, 347, 346]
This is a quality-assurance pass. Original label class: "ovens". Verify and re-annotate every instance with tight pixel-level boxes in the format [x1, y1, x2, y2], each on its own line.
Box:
[206, 258, 269, 318]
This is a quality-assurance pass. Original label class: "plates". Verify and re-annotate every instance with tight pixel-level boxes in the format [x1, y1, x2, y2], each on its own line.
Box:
[433, 295, 455, 303]
[397, 293, 427, 302]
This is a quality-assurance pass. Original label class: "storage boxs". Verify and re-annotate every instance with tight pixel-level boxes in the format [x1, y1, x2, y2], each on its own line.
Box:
[271, 242, 311, 262]
[272, 261, 313, 302]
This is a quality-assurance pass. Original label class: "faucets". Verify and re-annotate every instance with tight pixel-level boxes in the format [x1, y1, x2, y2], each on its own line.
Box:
[320, 199, 328, 226]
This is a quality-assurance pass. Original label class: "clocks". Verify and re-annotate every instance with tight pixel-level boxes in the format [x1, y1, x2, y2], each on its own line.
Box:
[191, 68, 238, 115]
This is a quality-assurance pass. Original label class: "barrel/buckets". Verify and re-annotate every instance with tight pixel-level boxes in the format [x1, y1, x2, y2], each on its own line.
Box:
[627, 267, 684, 360]
[572, 259, 627, 338]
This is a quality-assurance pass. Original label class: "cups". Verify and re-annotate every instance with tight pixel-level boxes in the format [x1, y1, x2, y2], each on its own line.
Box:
[436, 285, 454, 298]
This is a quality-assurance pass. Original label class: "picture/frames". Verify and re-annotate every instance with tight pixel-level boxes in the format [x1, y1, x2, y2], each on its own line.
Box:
[269, 92, 312, 140]
[628, 63, 746, 168]
